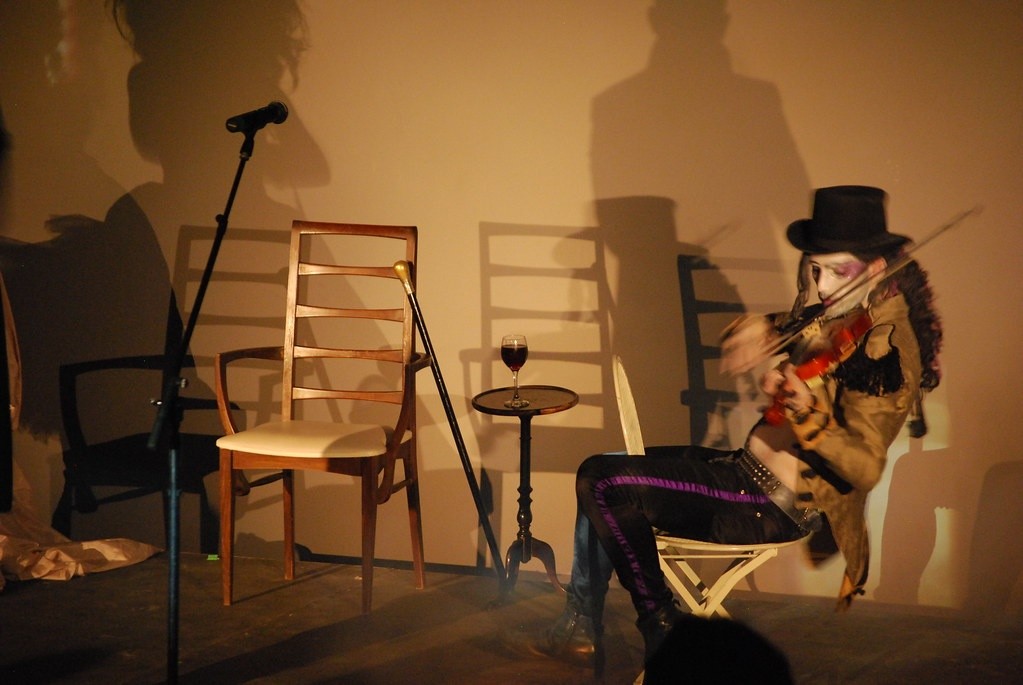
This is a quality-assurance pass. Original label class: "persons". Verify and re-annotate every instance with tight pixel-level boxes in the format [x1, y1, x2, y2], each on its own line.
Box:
[643, 616, 792, 685]
[496, 185, 944, 685]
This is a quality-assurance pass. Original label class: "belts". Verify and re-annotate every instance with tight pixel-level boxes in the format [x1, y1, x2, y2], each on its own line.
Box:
[734, 447, 816, 529]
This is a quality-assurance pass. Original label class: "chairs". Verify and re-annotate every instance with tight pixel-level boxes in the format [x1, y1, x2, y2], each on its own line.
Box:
[212, 220, 432, 617]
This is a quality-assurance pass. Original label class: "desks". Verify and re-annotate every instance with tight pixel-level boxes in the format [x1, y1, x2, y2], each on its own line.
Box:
[472, 385, 579, 606]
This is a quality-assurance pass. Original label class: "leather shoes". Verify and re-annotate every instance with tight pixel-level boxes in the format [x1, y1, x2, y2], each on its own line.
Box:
[635, 599, 683, 667]
[495, 606, 605, 677]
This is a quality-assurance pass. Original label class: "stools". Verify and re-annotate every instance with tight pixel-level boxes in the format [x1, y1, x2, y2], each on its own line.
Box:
[628, 527, 815, 685]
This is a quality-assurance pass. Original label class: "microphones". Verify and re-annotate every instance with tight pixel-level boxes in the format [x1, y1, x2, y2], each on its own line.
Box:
[226, 100, 289, 133]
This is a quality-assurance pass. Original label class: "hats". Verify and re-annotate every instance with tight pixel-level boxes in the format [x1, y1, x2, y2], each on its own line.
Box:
[786, 186, 910, 254]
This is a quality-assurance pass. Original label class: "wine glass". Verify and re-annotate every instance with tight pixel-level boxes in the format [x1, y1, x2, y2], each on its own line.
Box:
[500, 334, 529, 408]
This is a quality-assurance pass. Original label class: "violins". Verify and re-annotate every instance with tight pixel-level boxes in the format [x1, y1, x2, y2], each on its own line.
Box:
[764, 304, 873, 426]
